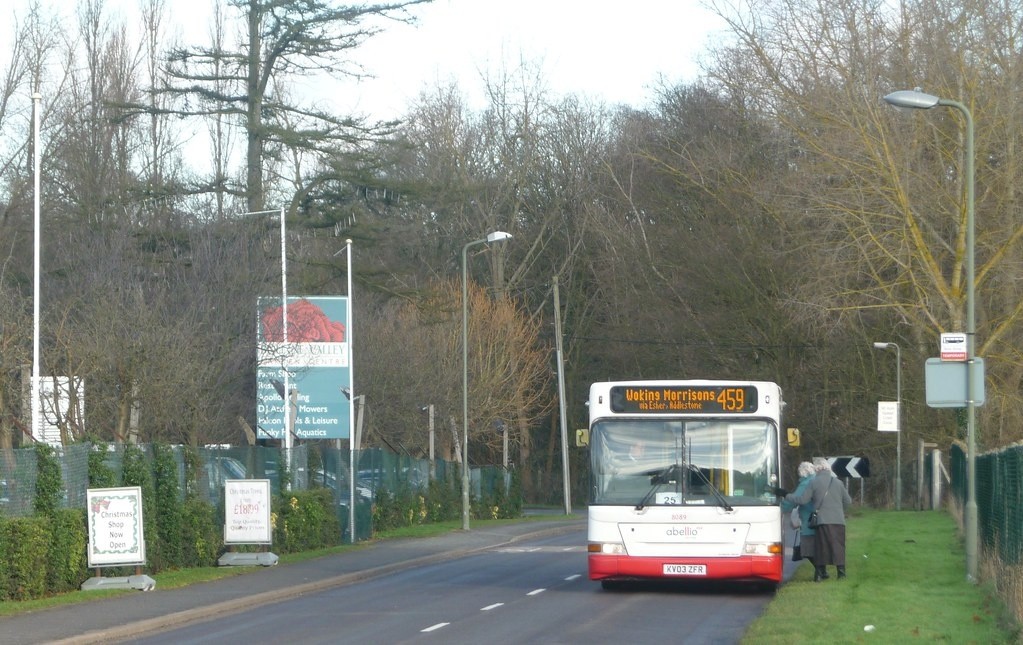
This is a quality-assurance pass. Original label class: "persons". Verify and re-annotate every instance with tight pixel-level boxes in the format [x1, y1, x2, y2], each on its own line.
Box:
[783, 456, 852, 579]
[780, 460, 830, 582]
[607, 437, 646, 491]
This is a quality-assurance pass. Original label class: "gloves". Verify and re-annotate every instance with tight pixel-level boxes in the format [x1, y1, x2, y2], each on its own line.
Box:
[773, 487, 788, 497]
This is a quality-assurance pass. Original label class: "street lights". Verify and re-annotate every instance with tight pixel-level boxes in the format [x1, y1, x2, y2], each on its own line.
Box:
[881, 85, 981, 588]
[459, 231, 514, 531]
[877, 340, 904, 511]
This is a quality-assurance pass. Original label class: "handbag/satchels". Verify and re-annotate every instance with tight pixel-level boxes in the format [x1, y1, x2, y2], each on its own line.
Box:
[790, 507, 801, 528]
[792, 529, 803, 560]
[808, 511, 817, 528]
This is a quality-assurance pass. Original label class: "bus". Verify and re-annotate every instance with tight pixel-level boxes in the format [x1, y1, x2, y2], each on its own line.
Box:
[574, 378, 802, 593]
